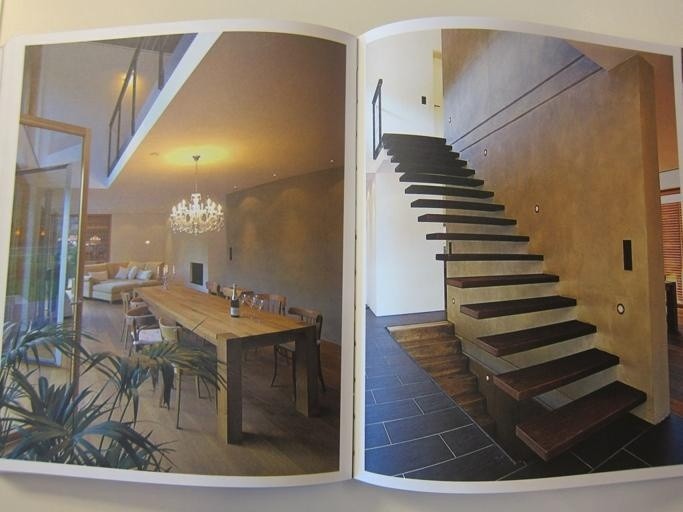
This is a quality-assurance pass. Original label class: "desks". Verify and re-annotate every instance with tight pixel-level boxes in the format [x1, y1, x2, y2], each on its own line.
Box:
[134, 283, 319, 444]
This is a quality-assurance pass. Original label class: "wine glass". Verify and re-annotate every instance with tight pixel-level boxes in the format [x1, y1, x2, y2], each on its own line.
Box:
[242, 293, 265, 319]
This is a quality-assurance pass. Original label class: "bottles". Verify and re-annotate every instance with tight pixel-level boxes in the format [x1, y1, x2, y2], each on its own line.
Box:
[230, 283, 240, 317]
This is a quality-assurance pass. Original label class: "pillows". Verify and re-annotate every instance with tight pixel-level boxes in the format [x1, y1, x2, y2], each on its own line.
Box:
[86, 264, 154, 283]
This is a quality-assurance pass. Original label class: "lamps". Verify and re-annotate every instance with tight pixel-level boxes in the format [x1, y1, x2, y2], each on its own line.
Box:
[167, 153, 226, 235]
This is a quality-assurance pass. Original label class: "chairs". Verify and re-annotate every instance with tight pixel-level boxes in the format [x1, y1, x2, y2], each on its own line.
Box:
[117, 280, 327, 430]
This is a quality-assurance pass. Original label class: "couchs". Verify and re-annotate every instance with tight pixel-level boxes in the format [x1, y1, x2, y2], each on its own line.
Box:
[80, 260, 164, 305]
